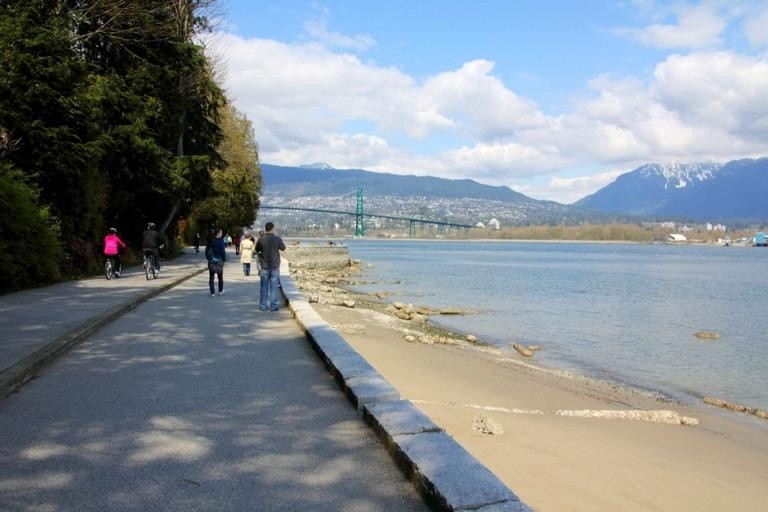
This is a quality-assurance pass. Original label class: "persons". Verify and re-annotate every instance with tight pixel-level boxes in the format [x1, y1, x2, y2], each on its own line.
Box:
[193, 231, 255, 276]
[204, 226, 226, 297]
[255, 222, 286, 311]
[142, 223, 166, 273]
[104, 227, 127, 277]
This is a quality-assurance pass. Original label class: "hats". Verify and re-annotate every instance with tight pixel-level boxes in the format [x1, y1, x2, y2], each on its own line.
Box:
[110, 228, 116, 233]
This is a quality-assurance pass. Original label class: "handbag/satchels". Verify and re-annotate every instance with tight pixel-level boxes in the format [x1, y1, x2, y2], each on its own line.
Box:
[209, 256, 224, 273]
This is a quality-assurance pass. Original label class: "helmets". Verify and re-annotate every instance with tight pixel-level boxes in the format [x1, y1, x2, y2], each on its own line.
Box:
[147, 223, 156, 229]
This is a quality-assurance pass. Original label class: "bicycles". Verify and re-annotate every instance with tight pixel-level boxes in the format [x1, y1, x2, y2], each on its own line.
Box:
[144, 246, 163, 280]
[104, 256, 122, 280]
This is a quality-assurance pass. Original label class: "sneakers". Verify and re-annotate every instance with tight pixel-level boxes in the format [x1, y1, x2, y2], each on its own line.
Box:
[210, 289, 225, 297]
[155, 269, 160, 273]
[115, 271, 120, 277]
